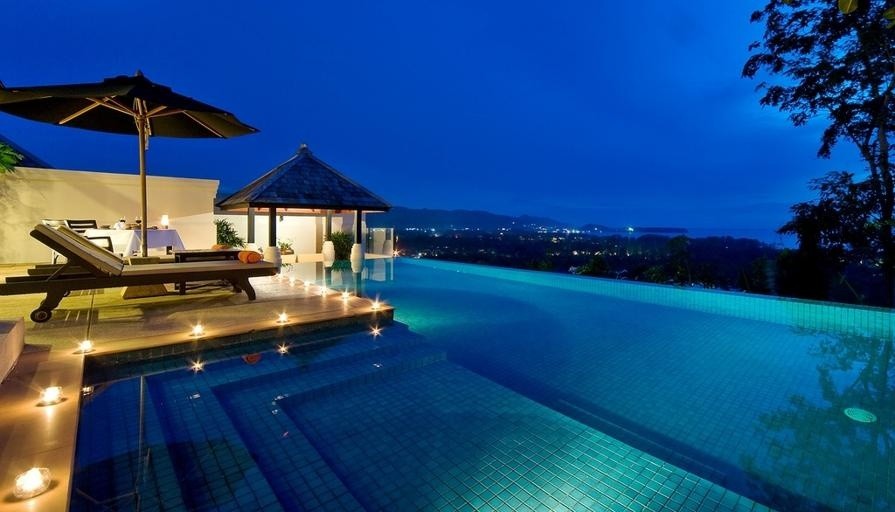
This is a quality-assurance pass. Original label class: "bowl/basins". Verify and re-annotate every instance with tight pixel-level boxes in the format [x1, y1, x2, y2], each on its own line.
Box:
[81, 340, 94, 351]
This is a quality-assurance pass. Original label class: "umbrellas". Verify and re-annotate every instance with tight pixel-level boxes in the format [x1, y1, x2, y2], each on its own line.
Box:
[2, 69, 263, 259]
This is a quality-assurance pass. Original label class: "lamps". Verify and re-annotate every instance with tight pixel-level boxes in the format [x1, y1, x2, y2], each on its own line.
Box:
[161, 215, 168, 229]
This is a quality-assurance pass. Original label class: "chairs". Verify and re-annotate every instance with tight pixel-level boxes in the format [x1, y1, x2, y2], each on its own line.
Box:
[0, 218, 280, 323]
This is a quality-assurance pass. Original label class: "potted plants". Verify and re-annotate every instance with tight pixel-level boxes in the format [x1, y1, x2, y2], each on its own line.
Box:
[325, 232, 354, 260]
[277, 241, 294, 255]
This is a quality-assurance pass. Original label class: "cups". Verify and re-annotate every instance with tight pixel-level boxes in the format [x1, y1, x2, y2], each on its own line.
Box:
[161, 214, 169, 229]
[120, 216, 126, 223]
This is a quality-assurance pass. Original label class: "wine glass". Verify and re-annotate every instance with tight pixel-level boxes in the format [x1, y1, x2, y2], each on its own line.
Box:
[135, 216, 142, 229]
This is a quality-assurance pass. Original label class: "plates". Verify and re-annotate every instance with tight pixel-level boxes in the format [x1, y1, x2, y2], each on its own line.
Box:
[99, 224, 110, 228]
